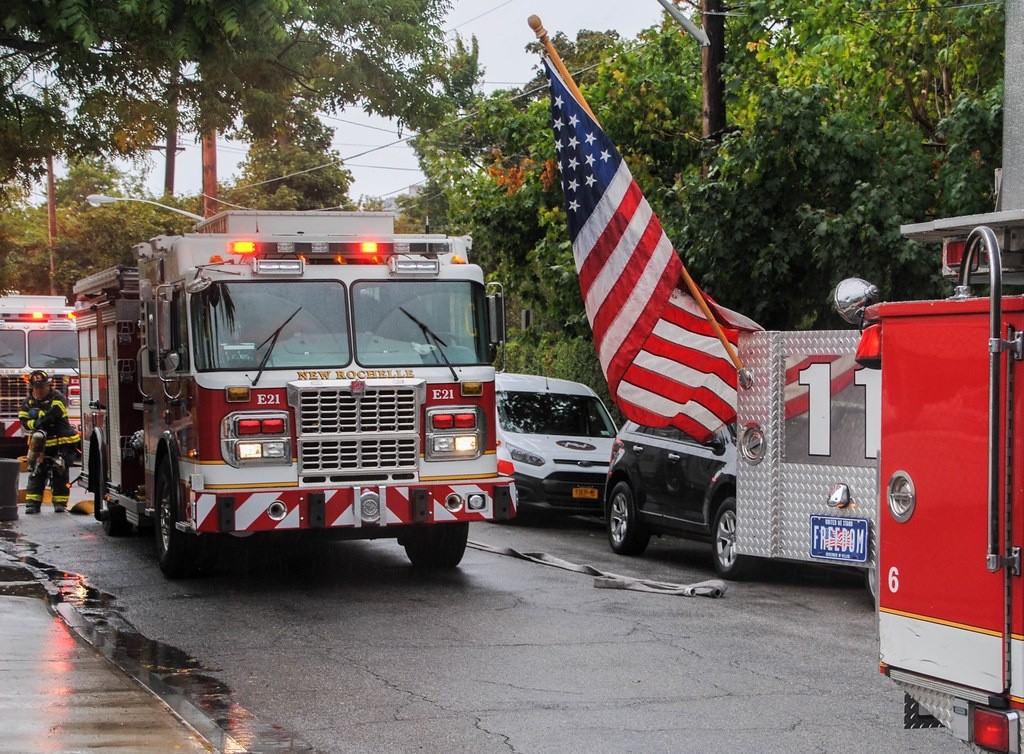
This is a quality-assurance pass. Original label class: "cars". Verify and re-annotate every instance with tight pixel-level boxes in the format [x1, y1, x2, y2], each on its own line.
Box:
[602, 411, 769, 583]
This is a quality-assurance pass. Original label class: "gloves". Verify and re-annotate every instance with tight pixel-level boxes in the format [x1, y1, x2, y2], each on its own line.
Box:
[33, 418, 46, 430]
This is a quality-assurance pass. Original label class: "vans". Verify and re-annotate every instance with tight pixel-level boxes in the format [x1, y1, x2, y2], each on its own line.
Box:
[497, 372, 627, 517]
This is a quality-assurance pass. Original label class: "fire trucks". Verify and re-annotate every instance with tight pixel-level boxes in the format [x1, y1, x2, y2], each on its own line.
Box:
[0, 293, 89, 463]
[733, 201, 1024, 754]
[71, 207, 521, 587]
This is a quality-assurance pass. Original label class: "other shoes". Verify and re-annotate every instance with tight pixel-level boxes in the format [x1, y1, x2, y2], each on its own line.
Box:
[24, 506, 40, 513]
[55, 505, 66, 512]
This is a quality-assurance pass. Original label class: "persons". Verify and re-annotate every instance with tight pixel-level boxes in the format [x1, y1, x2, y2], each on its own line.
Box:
[18, 370, 81, 514]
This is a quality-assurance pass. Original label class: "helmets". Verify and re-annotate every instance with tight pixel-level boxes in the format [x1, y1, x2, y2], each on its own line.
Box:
[27, 369, 53, 388]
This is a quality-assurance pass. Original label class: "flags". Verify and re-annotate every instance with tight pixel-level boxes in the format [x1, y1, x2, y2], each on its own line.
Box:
[542, 54, 765, 447]
[783, 355, 862, 420]
